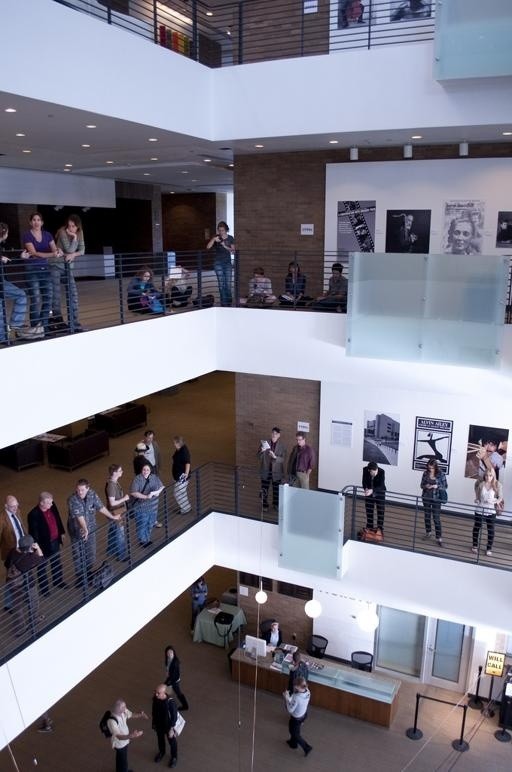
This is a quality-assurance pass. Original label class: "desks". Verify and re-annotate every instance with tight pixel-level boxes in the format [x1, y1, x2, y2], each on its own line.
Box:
[229, 644, 402, 729]
[196, 602, 242, 648]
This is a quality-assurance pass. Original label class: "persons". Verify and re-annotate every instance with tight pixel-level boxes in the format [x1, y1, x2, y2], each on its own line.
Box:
[128, 267, 159, 316]
[279, 262, 315, 308]
[263, 623, 282, 648]
[171, 435, 192, 514]
[163, 265, 193, 307]
[132, 464, 164, 547]
[0, 494, 30, 613]
[152, 684, 179, 769]
[164, 645, 189, 712]
[309, 264, 348, 313]
[0, 222, 31, 345]
[476, 439, 503, 480]
[416, 432, 449, 463]
[50, 214, 85, 332]
[445, 218, 477, 255]
[282, 676, 313, 758]
[362, 463, 386, 532]
[397, 214, 417, 253]
[288, 431, 315, 490]
[472, 467, 503, 557]
[133, 442, 153, 475]
[420, 459, 448, 546]
[206, 222, 236, 306]
[144, 430, 161, 527]
[36, 712, 54, 733]
[258, 427, 286, 512]
[249, 267, 276, 304]
[498, 221, 512, 244]
[289, 652, 308, 695]
[5, 535, 43, 634]
[25, 213, 64, 332]
[108, 699, 148, 771]
[26, 491, 71, 599]
[104, 463, 130, 562]
[190, 576, 208, 635]
[66, 479, 121, 589]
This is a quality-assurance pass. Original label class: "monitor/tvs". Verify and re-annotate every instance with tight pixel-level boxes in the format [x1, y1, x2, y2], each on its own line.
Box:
[245, 635, 266, 659]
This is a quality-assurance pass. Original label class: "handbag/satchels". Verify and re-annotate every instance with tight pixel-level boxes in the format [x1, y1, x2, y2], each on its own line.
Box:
[104, 481, 127, 518]
[495, 498, 506, 518]
[93, 560, 114, 589]
[361, 528, 382, 542]
[167, 698, 185, 737]
[441, 489, 449, 503]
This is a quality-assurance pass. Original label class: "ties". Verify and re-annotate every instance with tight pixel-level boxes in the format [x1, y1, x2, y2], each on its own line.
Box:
[11, 514, 23, 538]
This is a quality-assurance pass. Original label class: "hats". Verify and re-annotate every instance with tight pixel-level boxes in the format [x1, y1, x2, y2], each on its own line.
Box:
[19, 534, 34, 551]
[135, 443, 150, 454]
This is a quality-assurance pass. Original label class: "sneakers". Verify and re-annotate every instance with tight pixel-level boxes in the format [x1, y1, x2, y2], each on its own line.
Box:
[37, 724, 52, 733]
[191, 629, 194, 635]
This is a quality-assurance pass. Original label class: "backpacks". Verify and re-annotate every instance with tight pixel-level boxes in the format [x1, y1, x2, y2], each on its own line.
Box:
[100, 710, 118, 737]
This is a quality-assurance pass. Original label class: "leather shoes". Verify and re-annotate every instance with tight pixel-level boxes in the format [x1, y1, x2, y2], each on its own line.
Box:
[155, 521, 163, 528]
[169, 756, 179, 770]
[274, 505, 279, 512]
[53, 582, 71, 589]
[285, 739, 299, 750]
[31, 615, 45, 626]
[118, 556, 133, 562]
[436, 536, 443, 546]
[14, 625, 30, 637]
[342, 23, 350, 29]
[423, 534, 433, 541]
[41, 590, 52, 598]
[357, 18, 368, 27]
[180, 509, 193, 516]
[176, 511, 183, 515]
[305, 745, 313, 759]
[139, 541, 153, 549]
[485, 551, 494, 558]
[472, 548, 479, 555]
[263, 507, 270, 513]
[178, 704, 189, 711]
[154, 748, 169, 761]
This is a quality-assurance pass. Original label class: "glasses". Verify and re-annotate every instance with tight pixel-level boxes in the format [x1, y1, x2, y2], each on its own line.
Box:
[153, 689, 162, 697]
[295, 438, 305, 443]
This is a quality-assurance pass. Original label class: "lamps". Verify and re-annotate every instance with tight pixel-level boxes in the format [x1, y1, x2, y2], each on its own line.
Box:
[459, 138, 469, 156]
[350, 144, 358, 160]
[255, 576, 267, 605]
[304, 590, 323, 619]
[404, 142, 413, 158]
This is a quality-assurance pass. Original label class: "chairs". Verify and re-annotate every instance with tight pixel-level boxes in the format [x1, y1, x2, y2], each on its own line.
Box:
[304, 634, 329, 660]
[351, 650, 375, 672]
